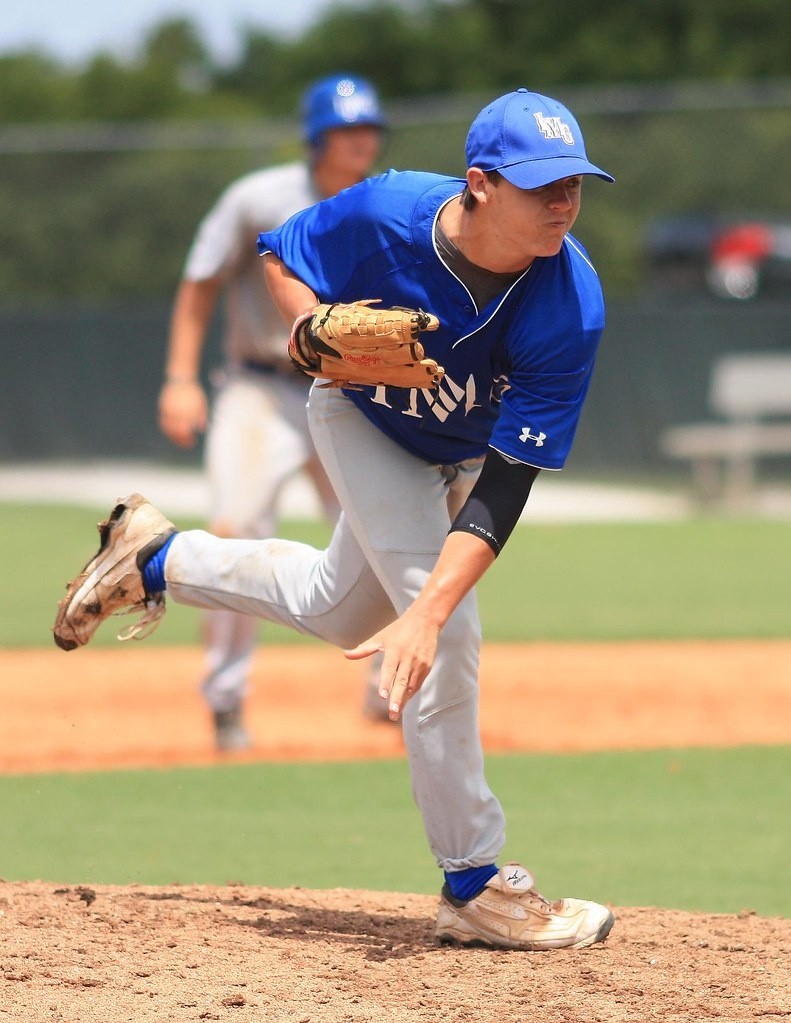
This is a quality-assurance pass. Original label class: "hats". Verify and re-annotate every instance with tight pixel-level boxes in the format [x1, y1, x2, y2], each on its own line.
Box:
[465, 88, 616, 190]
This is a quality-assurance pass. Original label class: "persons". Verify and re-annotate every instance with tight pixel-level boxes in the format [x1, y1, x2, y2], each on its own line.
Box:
[157, 70, 472, 753]
[53, 88, 616, 953]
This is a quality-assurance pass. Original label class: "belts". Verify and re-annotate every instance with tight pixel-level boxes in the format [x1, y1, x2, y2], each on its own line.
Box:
[243, 357, 278, 374]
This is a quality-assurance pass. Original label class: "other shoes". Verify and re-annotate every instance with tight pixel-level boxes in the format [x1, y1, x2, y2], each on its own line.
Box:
[216, 717, 251, 751]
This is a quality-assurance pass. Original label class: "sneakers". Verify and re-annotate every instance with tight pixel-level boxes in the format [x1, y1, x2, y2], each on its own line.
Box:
[435, 861, 616, 948]
[54, 495, 179, 651]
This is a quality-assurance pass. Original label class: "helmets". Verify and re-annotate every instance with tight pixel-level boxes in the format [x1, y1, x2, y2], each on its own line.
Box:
[300, 76, 388, 147]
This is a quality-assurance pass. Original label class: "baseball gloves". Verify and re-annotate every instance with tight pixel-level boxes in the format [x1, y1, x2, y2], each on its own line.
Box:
[282, 295, 449, 434]
